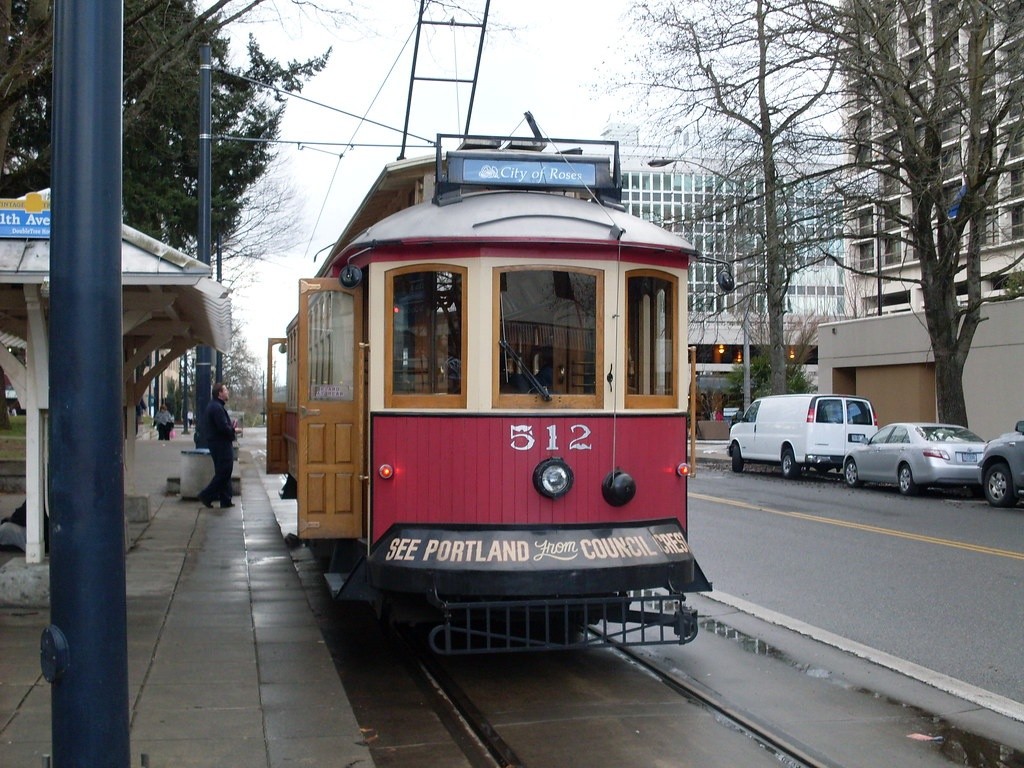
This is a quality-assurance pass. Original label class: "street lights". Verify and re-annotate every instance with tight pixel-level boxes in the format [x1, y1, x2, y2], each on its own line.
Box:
[646, 158, 752, 417]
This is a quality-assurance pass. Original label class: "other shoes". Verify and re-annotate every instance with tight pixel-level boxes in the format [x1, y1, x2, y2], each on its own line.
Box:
[221, 503, 236, 508]
[197, 492, 214, 508]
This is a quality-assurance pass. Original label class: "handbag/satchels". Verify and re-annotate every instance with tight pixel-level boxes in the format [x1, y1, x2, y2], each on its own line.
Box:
[169, 429, 175, 439]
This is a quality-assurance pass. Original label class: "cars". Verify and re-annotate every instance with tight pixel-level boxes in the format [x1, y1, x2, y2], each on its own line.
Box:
[976, 421, 1024, 509]
[843, 422, 988, 496]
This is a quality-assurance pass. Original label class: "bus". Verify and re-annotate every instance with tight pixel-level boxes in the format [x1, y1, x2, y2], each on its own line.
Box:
[265, 0, 736, 657]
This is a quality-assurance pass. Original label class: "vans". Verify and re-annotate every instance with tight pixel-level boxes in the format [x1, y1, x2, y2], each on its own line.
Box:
[726, 393, 877, 481]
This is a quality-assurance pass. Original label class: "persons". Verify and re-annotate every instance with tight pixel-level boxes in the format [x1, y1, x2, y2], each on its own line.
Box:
[447, 334, 461, 390]
[187, 408, 194, 428]
[123, 396, 148, 439]
[152, 405, 175, 441]
[197, 383, 236, 510]
[530, 344, 554, 392]
[0, 495, 50, 555]
[6, 401, 26, 417]
